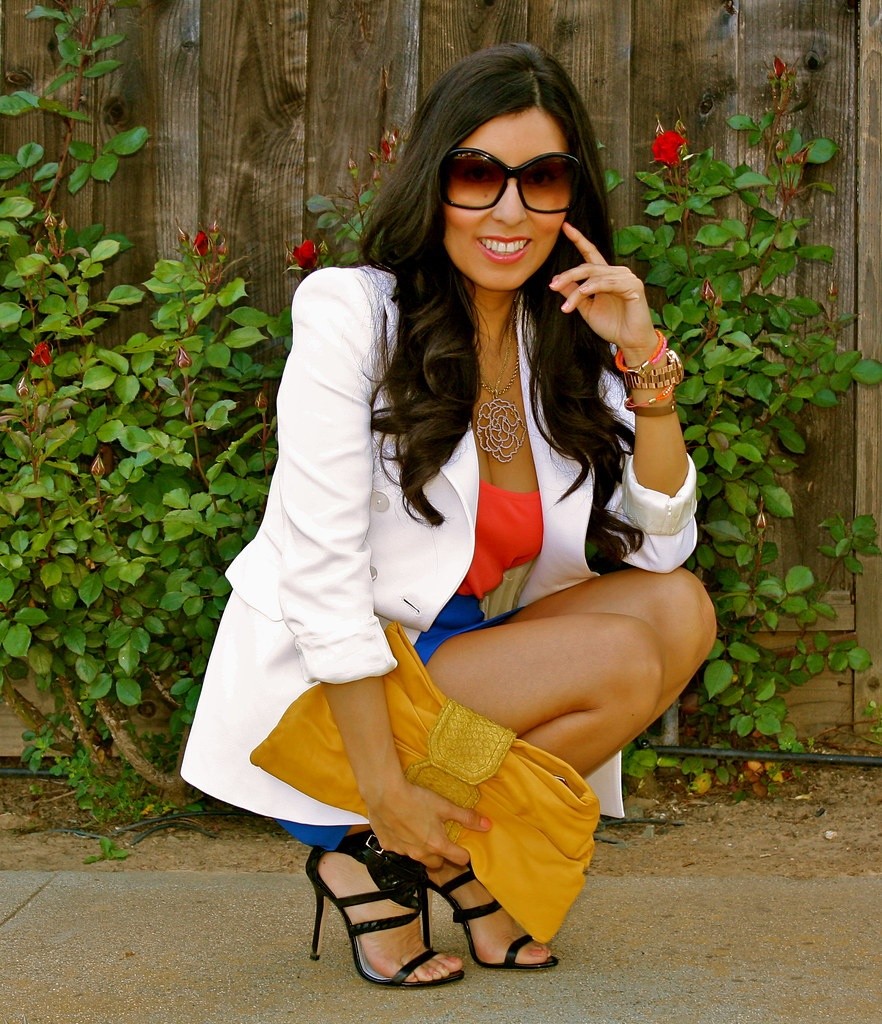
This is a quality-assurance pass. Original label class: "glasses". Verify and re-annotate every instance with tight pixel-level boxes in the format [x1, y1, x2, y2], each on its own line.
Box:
[439, 148, 582, 214]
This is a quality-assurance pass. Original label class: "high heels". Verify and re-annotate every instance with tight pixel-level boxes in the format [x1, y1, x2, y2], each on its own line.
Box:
[419, 864, 557, 969]
[305, 828, 463, 987]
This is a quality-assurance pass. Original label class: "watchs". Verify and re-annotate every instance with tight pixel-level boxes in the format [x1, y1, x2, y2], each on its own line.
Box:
[622, 348, 685, 388]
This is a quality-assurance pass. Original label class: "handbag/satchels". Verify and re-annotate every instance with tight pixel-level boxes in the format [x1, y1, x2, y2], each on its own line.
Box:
[251, 623, 601, 945]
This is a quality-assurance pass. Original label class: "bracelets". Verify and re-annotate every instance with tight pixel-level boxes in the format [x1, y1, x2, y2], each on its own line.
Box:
[622, 383, 675, 415]
[614, 331, 666, 376]
[621, 400, 679, 419]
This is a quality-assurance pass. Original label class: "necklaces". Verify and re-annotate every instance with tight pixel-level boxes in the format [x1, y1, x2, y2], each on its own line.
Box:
[474, 314, 528, 464]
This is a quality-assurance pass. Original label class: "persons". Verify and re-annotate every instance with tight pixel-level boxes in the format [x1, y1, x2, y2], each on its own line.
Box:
[178, 42, 718, 989]
